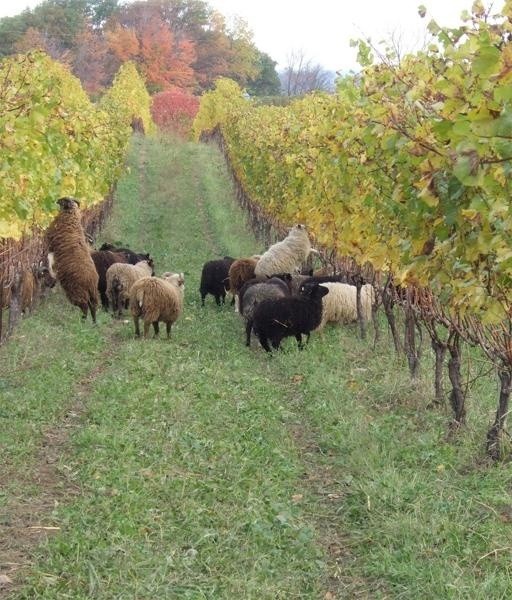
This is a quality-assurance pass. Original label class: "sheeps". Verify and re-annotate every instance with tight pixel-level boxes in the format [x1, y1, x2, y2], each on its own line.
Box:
[42, 195, 186, 341]
[198, 223, 382, 352]
